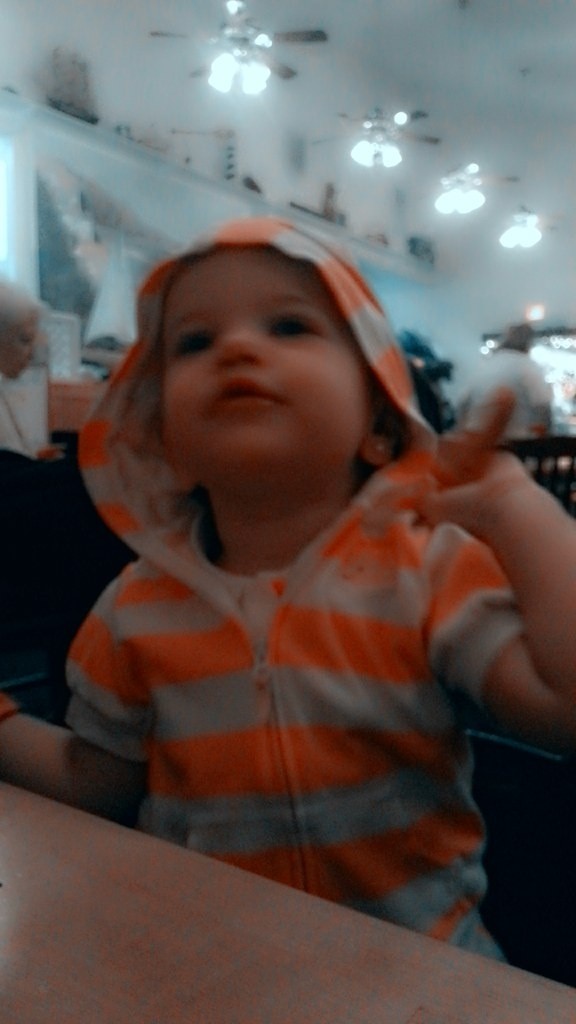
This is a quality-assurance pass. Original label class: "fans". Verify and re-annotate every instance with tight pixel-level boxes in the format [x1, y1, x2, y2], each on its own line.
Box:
[148, 0, 327, 82]
[309, 105, 440, 147]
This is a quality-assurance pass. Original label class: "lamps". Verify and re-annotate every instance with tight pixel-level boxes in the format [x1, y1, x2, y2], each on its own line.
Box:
[208, 37, 270, 97]
[350, 129, 402, 169]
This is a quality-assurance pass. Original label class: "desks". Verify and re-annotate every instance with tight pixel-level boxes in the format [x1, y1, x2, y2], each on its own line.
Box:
[0, 780, 576, 1024]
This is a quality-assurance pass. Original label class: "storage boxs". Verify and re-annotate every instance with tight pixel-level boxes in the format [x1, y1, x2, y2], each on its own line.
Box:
[47, 312, 83, 384]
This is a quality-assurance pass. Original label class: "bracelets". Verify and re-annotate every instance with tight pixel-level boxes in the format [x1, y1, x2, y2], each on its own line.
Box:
[0, 708, 22, 723]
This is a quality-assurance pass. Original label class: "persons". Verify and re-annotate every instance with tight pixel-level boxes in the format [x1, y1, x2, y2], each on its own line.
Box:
[0, 215, 576, 964]
[450, 320, 552, 438]
[0, 287, 48, 461]
